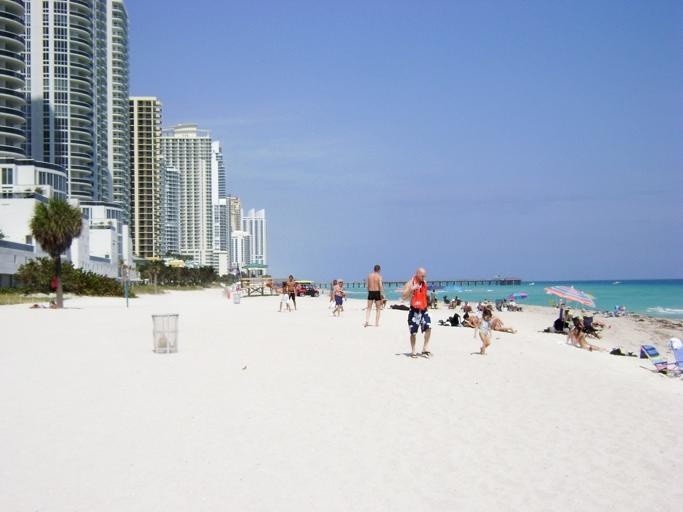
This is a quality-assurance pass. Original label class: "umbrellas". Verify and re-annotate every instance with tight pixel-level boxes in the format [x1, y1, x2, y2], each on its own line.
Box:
[580, 290, 597, 300]
[506, 291, 528, 301]
[543, 284, 595, 308]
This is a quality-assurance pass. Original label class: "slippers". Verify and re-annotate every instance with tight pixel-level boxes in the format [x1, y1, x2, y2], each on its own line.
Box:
[412, 351, 434, 359]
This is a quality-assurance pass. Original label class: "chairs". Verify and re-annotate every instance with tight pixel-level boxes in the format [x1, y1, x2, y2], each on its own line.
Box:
[640, 337, 683, 379]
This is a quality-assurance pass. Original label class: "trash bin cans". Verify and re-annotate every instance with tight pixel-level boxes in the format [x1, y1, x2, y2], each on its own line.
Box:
[234, 294, 240, 303]
[152, 315, 179, 354]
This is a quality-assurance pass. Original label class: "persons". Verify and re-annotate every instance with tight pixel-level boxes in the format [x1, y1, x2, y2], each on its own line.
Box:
[363, 295, 387, 311]
[427, 289, 523, 334]
[332, 279, 347, 316]
[474, 309, 492, 355]
[402, 267, 432, 358]
[555, 298, 626, 352]
[278, 282, 291, 312]
[286, 274, 297, 310]
[364, 265, 383, 326]
[329, 280, 344, 311]
